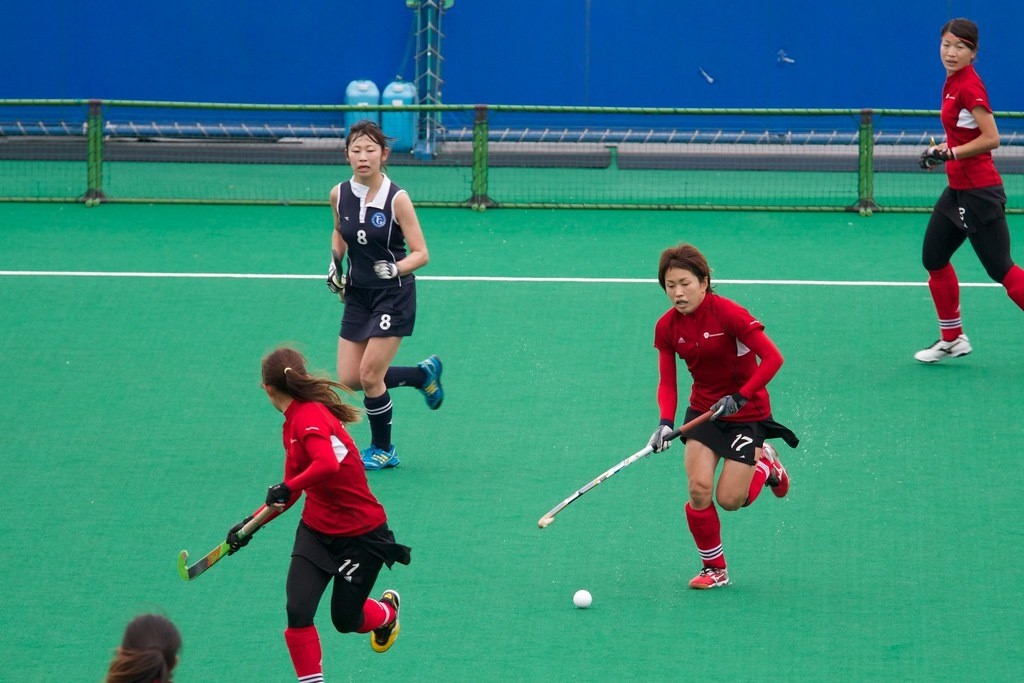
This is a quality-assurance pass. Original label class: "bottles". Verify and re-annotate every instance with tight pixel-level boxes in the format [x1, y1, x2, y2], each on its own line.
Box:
[344, 78, 381, 141]
[381, 81, 420, 154]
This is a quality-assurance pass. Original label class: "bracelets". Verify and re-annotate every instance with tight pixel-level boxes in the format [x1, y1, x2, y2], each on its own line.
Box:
[951, 147, 956, 160]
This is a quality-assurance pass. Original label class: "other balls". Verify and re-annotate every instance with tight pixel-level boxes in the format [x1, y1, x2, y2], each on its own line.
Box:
[572, 590, 593, 608]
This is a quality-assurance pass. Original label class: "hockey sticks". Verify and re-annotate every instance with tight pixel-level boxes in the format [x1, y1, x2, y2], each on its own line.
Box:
[331, 248, 344, 303]
[537, 409, 717, 529]
[176, 505, 276, 581]
[925, 136, 936, 168]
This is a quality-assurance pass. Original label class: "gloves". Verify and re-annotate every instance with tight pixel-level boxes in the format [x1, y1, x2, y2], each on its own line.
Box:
[645, 425, 675, 458]
[327, 263, 348, 293]
[708, 392, 745, 420]
[226, 517, 261, 556]
[919, 146, 957, 172]
[374, 260, 400, 279]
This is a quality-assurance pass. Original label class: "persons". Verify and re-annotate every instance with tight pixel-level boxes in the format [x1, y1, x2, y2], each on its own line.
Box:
[912, 20, 1024, 363]
[226, 348, 412, 683]
[327, 120, 442, 469]
[105, 615, 181, 683]
[646, 244, 799, 587]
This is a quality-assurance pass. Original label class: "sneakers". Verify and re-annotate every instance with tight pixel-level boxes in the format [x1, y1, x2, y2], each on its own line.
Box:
[371, 589, 400, 652]
[360, 445, 399, 470]
[761, 442, 789, 497]
[689, 567, 729, 588]
[418, 354, 443, 410]
[915, 333, 973, 363]
